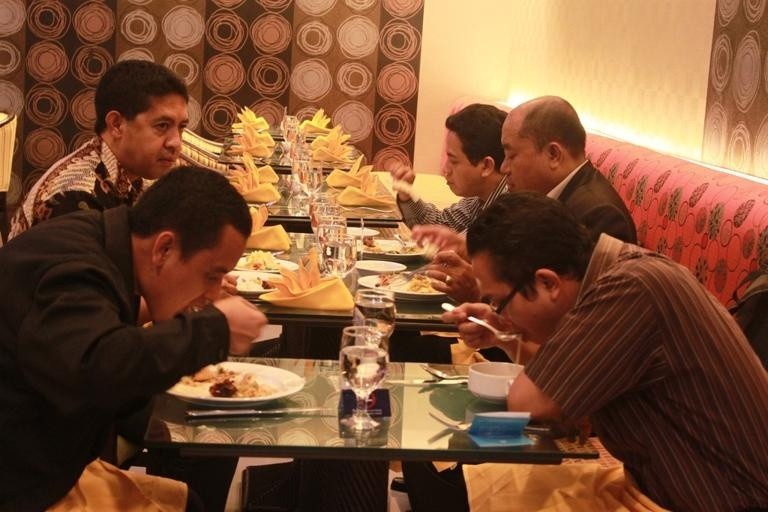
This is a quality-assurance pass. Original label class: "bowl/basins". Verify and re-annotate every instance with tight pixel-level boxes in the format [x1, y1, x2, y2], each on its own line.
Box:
[467, 359, 524, 402]
[354, 260, 408, 277]
[346, 226, 379, 238]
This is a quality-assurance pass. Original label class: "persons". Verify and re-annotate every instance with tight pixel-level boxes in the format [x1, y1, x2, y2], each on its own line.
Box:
[442, 191, 768, 512]
[0, 167, 269, 512]
[6, 60, 239, 512]
[391, 96, 636, 512]
[386, 103, 509, 249]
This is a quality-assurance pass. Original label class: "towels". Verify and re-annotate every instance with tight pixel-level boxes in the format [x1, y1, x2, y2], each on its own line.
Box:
[221, 105, 398, 254]
[256, 248, 355, 312]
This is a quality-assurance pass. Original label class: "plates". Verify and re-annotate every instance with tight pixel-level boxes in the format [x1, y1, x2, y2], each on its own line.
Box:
[151, 397, 303, 428]
[164, 361, 305, 407]
[357, 240, 428, 261]
[359, 274, 451, 304]
[221, 270, 283, 294]
[241, 247, 284, 257]
[233, 258, 299, 272]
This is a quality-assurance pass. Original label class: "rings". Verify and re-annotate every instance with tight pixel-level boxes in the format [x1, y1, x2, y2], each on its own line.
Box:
[445, 275, 454, 285]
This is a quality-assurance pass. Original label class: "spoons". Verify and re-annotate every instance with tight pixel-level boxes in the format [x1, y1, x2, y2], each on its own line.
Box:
[442, 303, 521, 342]
[418, 384, 443, 394]
[420, 364, 469, 379]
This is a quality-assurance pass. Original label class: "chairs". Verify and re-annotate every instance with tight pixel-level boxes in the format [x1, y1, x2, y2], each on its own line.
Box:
[0, 109, 19, 245]
[182, 126, 223, 155]
[144, 139, 223, 190]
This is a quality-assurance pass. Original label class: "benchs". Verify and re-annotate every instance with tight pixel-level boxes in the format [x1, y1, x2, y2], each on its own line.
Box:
[439, 96, 768, 469]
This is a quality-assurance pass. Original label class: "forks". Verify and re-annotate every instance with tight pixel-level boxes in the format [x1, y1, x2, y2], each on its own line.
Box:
[427, 412, 552, 432]
[390, 260, 442, 286]
[426, 429, 458, 444]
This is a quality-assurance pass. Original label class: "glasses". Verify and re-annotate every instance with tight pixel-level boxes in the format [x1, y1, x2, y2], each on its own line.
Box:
[490, 282, 520, 314]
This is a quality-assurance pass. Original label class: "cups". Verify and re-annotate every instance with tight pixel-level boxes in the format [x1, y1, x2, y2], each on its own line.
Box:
[351, 290, 398, 342]
[281, 115, 356, 278]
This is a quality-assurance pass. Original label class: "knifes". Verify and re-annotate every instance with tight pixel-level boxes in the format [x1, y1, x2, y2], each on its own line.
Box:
[187, 407, 332, 418]
[385, 377, 467, 386]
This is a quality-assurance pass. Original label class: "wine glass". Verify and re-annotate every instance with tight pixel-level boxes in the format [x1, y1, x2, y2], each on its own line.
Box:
[339, 326, 388, 431]
